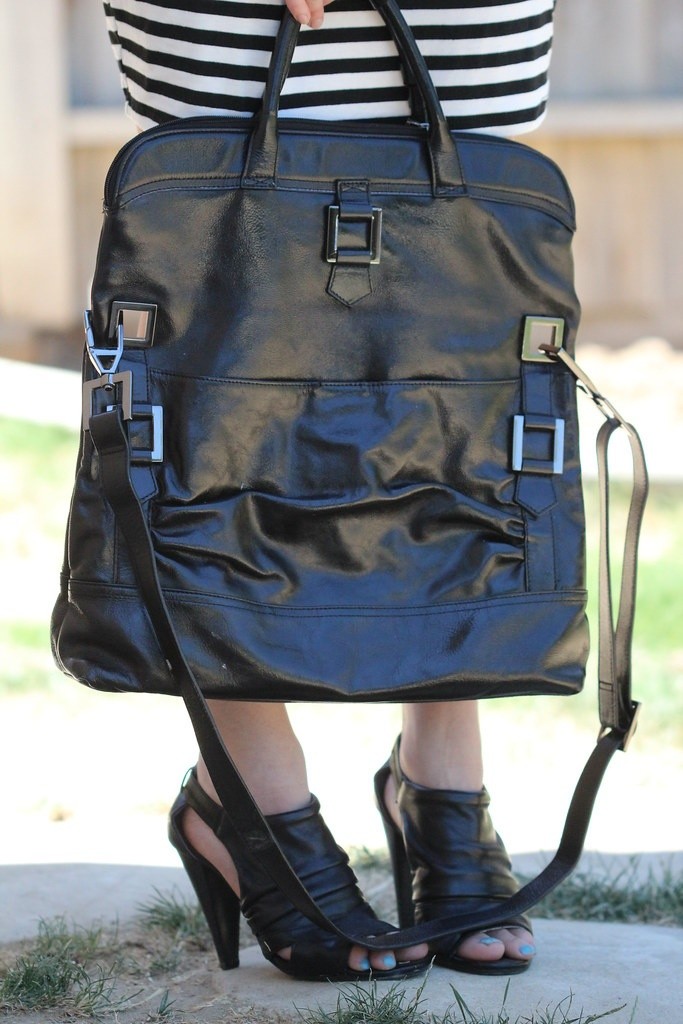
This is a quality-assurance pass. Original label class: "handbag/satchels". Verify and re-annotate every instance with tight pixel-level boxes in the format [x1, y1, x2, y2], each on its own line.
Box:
[46, 6, 591, 703]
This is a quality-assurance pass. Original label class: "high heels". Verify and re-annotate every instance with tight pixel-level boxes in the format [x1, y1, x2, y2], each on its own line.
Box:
[166, 766, 434, 983]
[373, 733, 536, 974]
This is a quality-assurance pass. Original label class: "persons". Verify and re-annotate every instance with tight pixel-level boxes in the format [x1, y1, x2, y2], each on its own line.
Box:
[104, 1, 560, 988]
